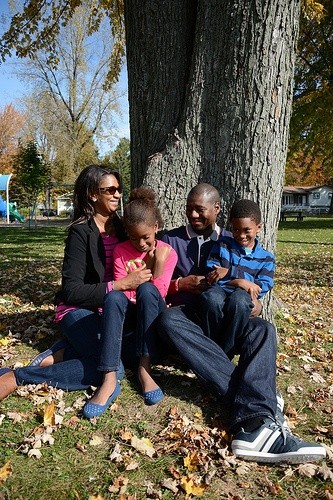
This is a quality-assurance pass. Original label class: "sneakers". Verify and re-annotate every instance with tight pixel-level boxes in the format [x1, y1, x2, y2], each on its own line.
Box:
[275, 392, 287, 430]
[231, 416, 326, 463]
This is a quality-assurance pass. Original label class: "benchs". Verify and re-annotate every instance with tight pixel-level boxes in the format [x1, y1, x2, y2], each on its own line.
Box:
[282, 215, 306, 219]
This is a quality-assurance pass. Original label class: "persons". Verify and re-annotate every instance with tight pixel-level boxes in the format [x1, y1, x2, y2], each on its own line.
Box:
[83, 188, 178, 419]
[155, 184, 326, 464]
[198, 199, 275, 362]
[0, 165, 152, 400]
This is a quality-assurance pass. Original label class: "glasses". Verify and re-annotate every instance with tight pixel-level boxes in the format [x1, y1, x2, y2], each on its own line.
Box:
[98, 185, 122, 194]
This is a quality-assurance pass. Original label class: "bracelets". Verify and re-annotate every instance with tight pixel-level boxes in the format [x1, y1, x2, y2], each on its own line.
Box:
[112, 280, 114, 290]
[175, 277, 182, 291]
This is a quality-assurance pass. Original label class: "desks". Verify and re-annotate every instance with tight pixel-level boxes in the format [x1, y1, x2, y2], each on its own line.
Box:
[281, 211, 303, 222]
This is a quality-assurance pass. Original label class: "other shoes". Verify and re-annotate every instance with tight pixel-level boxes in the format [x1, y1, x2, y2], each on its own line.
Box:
[28, 339, 68, 366]
[83, 382, 120, 418]
[0, 367, 12, 379]
[144, 387, 165, 405]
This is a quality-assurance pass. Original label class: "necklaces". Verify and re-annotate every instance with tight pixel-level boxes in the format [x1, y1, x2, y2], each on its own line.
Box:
[95, 217, 112, 237]
